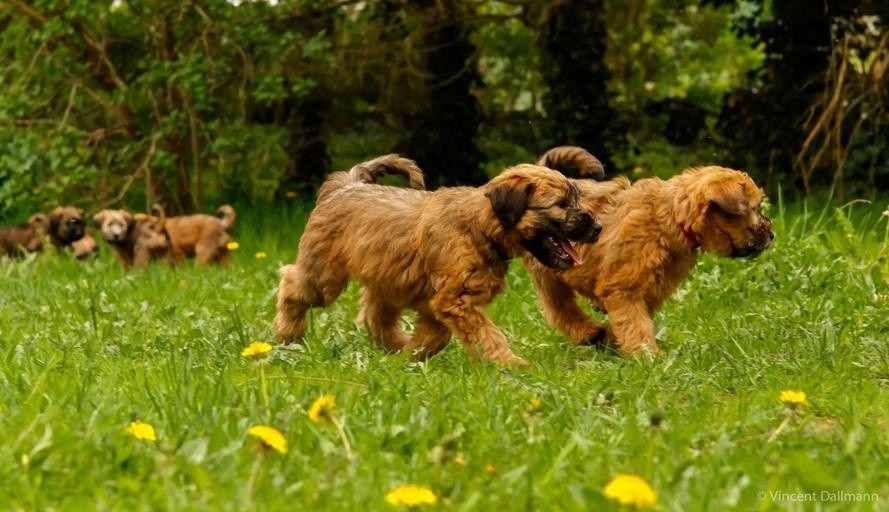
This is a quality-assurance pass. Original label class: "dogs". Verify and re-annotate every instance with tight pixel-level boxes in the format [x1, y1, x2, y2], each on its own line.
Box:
[274, 152, 602, 368]
[0, 204, 86, 258]
[163, 204, 236, 269]
[28, 212, 98, 260]
[521, 146, 775, 362]
[93, 203, 178, 279]
